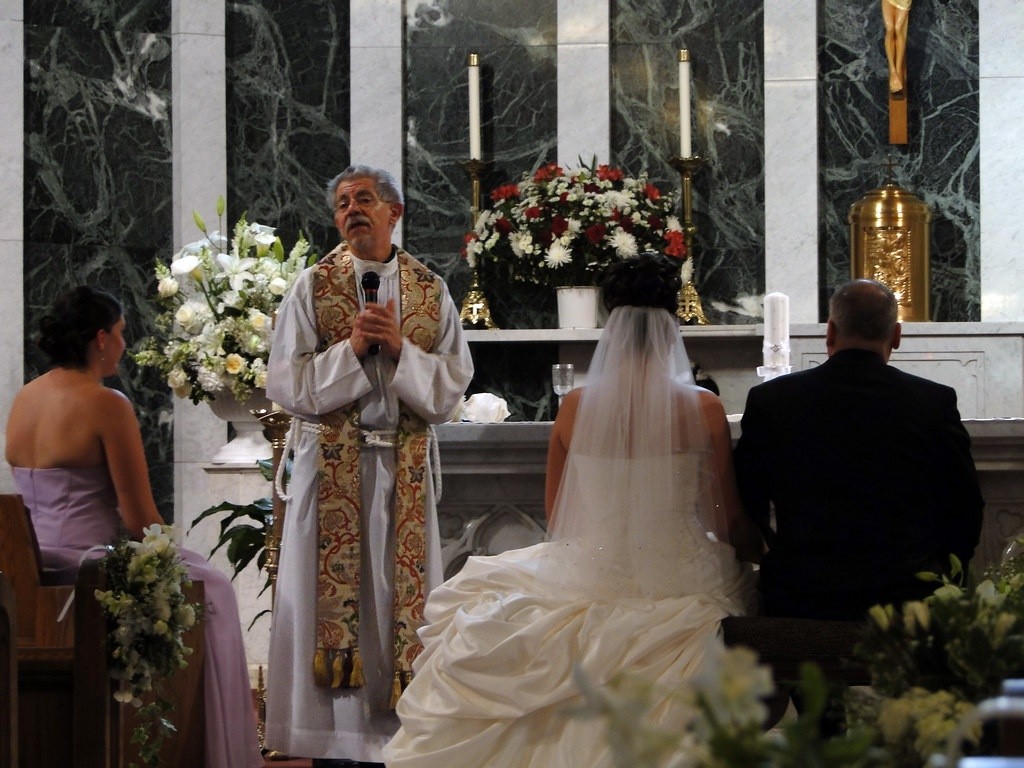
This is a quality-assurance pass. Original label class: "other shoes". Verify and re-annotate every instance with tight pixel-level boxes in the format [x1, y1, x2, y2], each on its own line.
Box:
[312, 758, 385, 768]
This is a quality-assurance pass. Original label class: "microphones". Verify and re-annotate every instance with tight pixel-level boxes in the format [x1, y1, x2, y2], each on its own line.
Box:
[361, 271, 380, 356]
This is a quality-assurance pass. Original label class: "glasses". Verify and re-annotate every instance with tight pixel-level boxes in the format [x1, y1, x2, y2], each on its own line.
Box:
[332, 192, 393, 216]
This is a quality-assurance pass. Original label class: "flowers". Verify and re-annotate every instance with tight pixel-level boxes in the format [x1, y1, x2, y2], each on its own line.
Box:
[93, 516, 205, 768]
[682, 529, 1024, 768]
[462, 153, 695, 286]
[133, 196, 317, 406]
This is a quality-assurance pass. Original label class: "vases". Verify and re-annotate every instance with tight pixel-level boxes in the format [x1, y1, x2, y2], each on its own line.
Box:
[554, 285, 601, 329]
[206, 387, 274, 464]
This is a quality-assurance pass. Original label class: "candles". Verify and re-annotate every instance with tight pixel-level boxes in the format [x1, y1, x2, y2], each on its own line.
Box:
[763, 291, 790, 366]
[677, 48, 691, 159]
[467, 52, 482, 161]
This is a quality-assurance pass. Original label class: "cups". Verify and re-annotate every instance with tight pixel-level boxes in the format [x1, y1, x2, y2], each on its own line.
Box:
[552, 362, 575, 396]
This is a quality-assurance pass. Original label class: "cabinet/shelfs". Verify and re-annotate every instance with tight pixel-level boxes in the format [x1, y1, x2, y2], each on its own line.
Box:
[431, 323, 764, 541]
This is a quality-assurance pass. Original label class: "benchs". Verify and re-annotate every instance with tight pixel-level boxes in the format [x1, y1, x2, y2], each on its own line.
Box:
[0, 493, 205, 768]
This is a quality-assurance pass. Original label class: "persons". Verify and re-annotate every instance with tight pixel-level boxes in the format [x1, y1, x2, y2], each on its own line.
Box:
[266, 164, 475, 761]
[4, 287, 262, 767]
[544, 252, 760, 617]
[729, 276, 984, 627]
